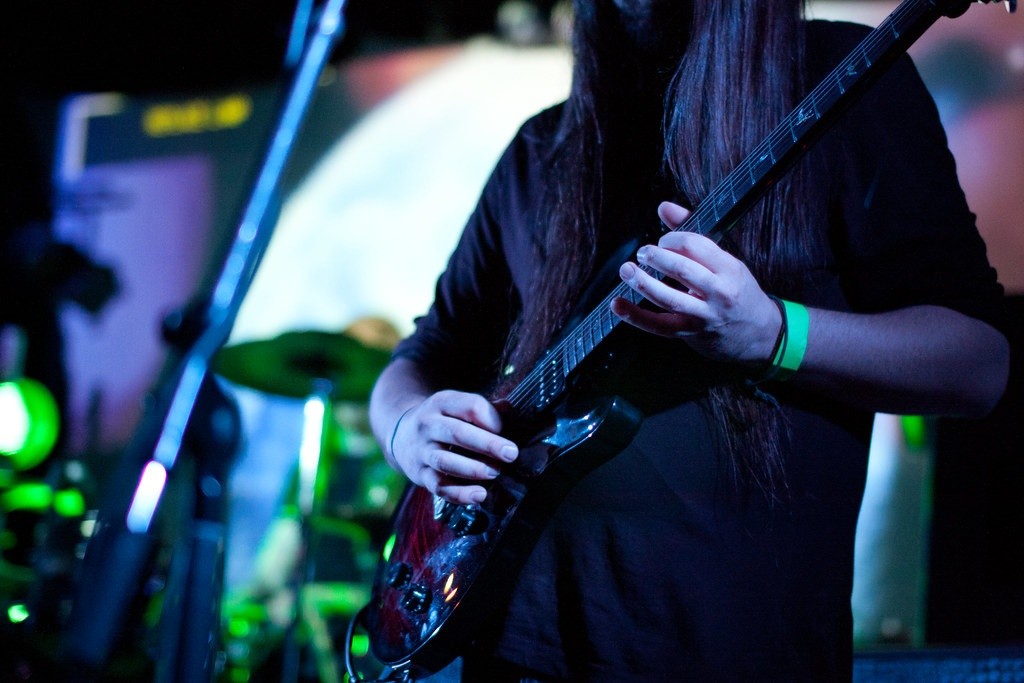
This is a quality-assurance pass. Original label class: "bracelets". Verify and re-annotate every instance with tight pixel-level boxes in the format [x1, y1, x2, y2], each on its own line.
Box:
[390, 406, 415, 456]
[768, 293, 810, 380]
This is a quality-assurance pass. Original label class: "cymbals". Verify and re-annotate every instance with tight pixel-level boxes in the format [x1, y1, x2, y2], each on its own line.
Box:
[211, 329, 396, 408]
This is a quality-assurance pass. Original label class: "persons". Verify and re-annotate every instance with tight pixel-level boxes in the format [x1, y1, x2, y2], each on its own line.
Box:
[347, 316, 399, 349]
[369, 1, 1014, 683]
[156, 303, 244, 522]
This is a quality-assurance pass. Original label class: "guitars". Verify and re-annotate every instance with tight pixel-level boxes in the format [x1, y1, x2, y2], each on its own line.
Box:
[360, 0, 1019, 683]
